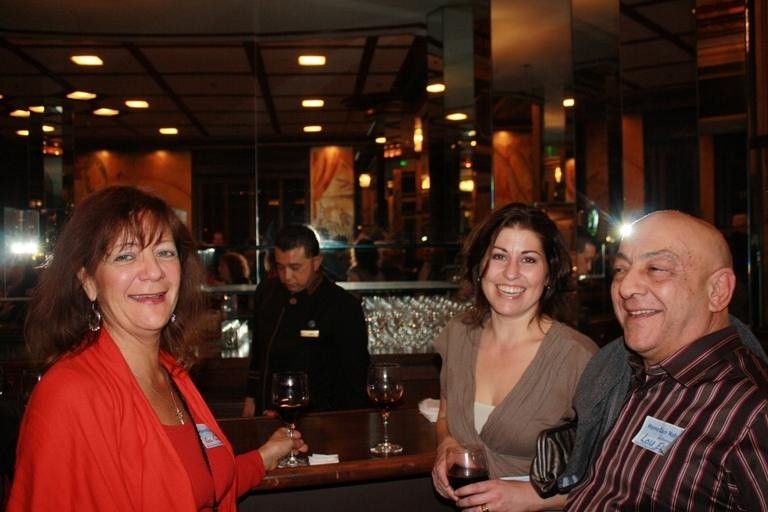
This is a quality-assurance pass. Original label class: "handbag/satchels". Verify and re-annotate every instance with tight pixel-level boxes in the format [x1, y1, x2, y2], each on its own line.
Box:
[529, 424, 578, 500]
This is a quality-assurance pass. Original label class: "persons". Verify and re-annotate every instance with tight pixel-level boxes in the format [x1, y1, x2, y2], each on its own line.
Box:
[562, 225, 600, 278]
[528, 308, 766, 499]
[429, 201, 603, 512]
[560, 209, 768, 511]
[240, 223, 372, 418]
[348, 236, 387, 281]
[4, 181, 308, 511]
[215, 251, 252, 321]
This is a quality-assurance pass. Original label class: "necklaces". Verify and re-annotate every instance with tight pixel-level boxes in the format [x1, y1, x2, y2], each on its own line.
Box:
[129, 366, 187, 425]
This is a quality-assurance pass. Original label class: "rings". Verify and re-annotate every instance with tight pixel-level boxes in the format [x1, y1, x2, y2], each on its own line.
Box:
[482, 504, 490, 511]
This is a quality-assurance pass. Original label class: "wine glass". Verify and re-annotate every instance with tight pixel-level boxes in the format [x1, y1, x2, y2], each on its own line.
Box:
[272, 370, 310, 469]
[364, 293, 475, 351]
[366, 363, 403, 453]
[445, 445, 490, 493]
